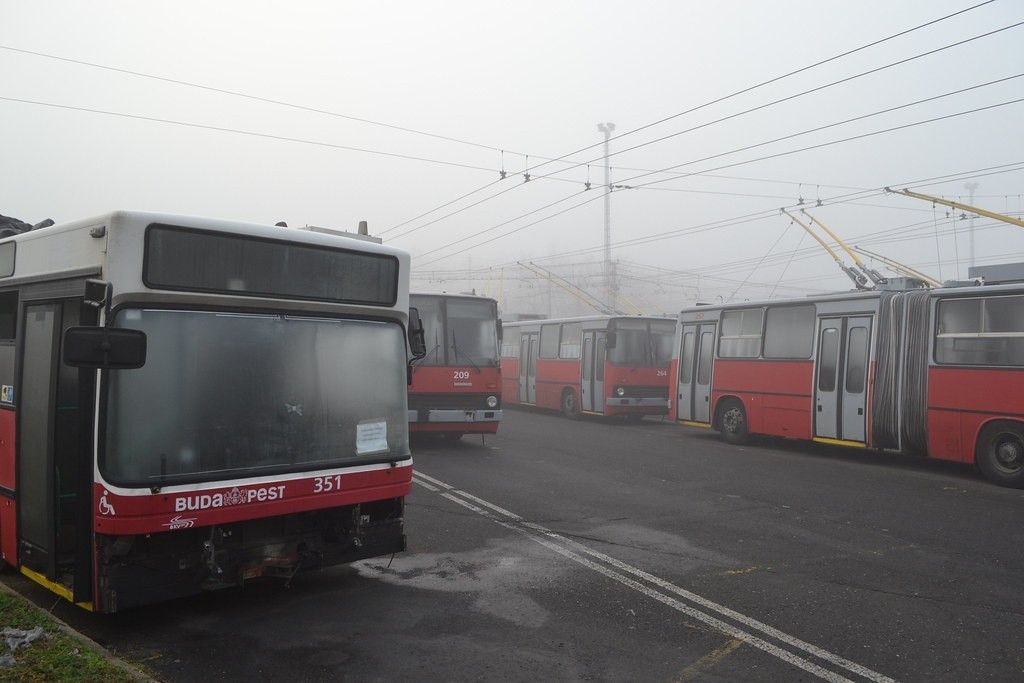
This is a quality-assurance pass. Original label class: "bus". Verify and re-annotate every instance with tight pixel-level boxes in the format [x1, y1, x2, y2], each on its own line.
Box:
[0, 209, 427, 618]
[406, 288, 504, 444]
[500, 260, 680, 421]
[670, 210, 1024, 486]
